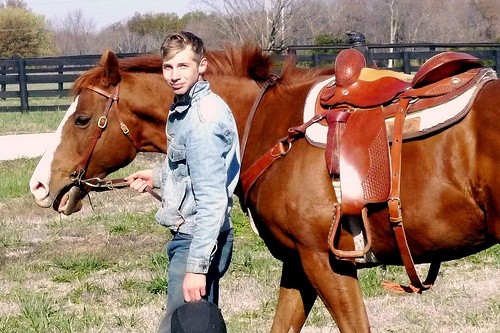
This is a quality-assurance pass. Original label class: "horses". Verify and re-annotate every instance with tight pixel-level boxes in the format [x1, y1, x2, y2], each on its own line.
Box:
[28, 37, 500, 333]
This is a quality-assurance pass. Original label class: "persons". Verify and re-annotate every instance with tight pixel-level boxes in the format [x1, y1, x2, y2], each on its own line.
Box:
[346, 32, 379, 70]
[123, 31, 241, 333]
